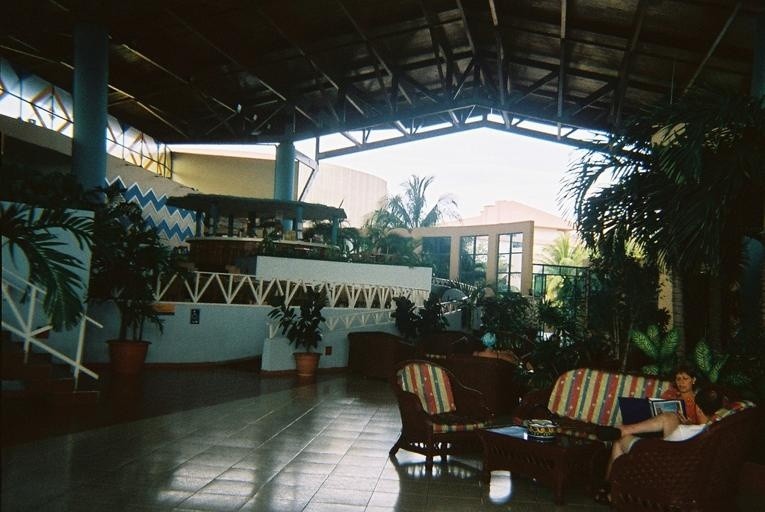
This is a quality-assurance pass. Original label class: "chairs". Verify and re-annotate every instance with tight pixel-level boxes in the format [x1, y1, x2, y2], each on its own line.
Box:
[606, 396, 753, 510]
[389, 357, 494, 469]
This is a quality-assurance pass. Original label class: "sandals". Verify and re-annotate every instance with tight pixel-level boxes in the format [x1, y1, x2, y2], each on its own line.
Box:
[595, 427, 622, 441]
[594, 489, 613, 504]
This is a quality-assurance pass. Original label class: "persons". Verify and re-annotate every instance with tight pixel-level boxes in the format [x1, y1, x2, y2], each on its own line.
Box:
[659, 365, 700, 426]
[472, 331, 521, 366]
[592, 388, 722, 504]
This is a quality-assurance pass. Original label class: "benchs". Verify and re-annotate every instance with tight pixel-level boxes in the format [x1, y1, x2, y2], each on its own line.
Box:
[513, 366, 738, 451]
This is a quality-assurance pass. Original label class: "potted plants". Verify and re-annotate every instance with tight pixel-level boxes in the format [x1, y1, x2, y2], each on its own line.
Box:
[268, 286, 329, 377]
[89, 203, 197, 376]
